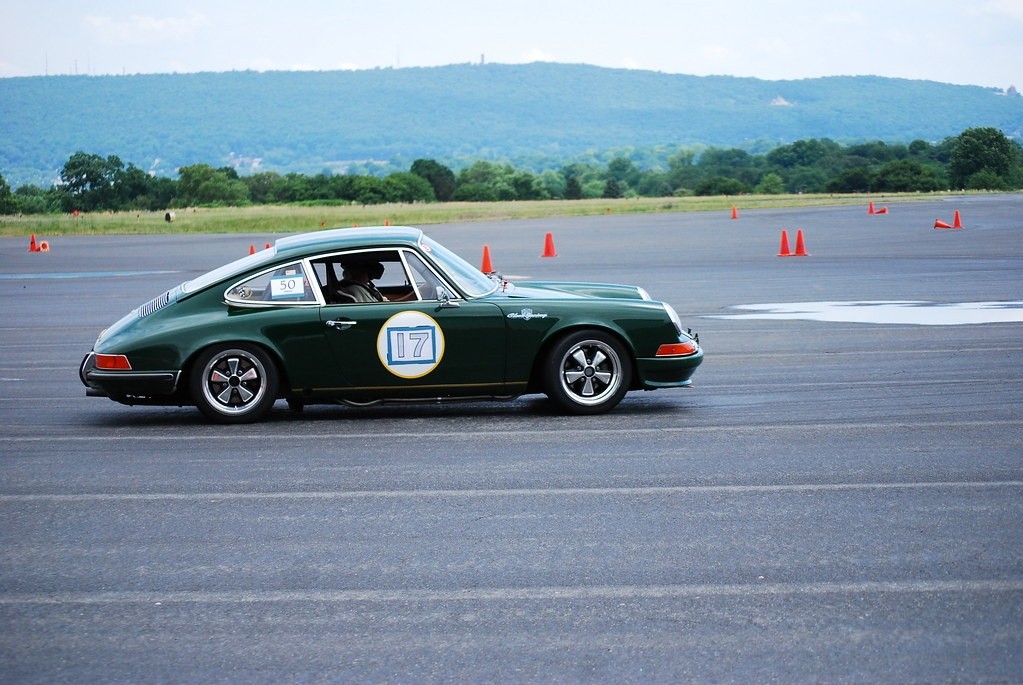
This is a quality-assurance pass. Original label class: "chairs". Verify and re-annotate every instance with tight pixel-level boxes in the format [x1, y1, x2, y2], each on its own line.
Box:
[335, 280, 358, 303]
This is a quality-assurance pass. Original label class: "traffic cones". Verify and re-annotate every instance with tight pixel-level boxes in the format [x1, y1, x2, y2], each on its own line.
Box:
[777, 229, 792, 256]
[265, 242, 270, 249]
[249, 244, 256, 255]
[953, 210, 963, 230]
[479, 244, 496, 274]
[876, 206, 887, 215]
[933, 217, 953, 229]
[36, 241, 48, 253]
[27, 234, 37, 252]
[541, 232, 558, 258]
[868, 201, 875, 214]
[729, 204, 739, 220]
[791, 229, 809, 256]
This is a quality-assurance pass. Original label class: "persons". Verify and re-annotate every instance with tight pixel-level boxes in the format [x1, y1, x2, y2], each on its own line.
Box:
[336, 258, 427, 302]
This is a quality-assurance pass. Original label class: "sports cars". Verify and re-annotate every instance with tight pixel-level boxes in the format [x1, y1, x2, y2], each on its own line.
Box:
[78, 225, 704, 426]
